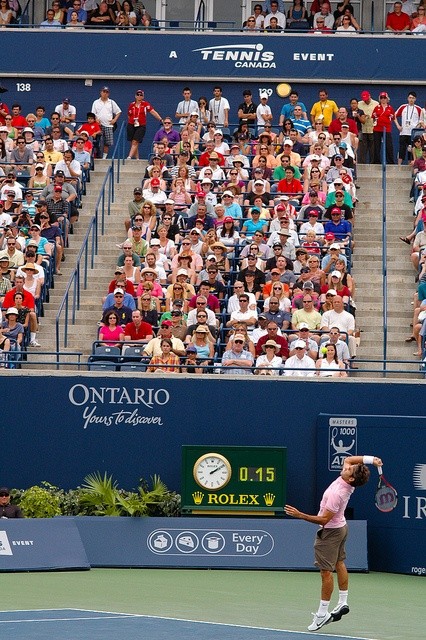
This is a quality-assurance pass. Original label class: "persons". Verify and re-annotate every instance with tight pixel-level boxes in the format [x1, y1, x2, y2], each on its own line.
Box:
[226, 144, 249, 167]
[294, 265, 320, 297]
[133, 295, 158, 330]
[227, 283, 258, 315]
[321, 243, 348, 273]
[304, 167, 328, 195]
[185, 296, 217, 329]
[242, 245, 264, 271]
[303, 181, 326, 206]
[16, 226, 28, 245]
[202, 228, 224, 258]
[227, 294, 258, 344]
[0, 223, 25, 253]
[18, 128, 39, 152]
[274, 195, 297, 220]
[72, 131, 92, 157]
[270, 257, 294, 285]
[331, 143, 355, 170]
[180, 229, 203, 260]
[291, 281, 320, 313]
[180, 349, 206, 374]
[264, 282, 291, 330]
[17, 251, 45, 286]
[116, 240, 142, 276]
[225, 324, 256, 362]
[0, 238, 24, 266]
[325, 178, 353, 209]
[259, 120, 276, 142]
[127, 188, 156, 217]
[227, 156, 249, 181]
[199, 256, 223, 285]
[281, 454, 385, 632]
[196, 266, 223, 298]
[0, 126, 13, 152]
[122, 310, 152, 357]
[167, 271, 197, 298]
[289, 323, 318, 360]
[0, 173, 22, 201]
[290, 295, 322, 344]
[238, 94, 274, 130]
[144, 166, 167, 191]
[189, 191, 213, 216]
[0, 0, 18, 28]
[137, 269, 164, 299]
[279, 119, 307, 144]
[0, 165, 6, 185]
[19, 188, 38, 220]
[321, 286, 345, 316]
[240, 232, 268, 260]
[99, 311, 122, 349]
[189, 217, 207, 240]
[155, 136, 174, 158]
[222, 168, 246, 194]
[171, 254, 195, 285]
[13, 209, 33, 227]
[220, 183, 242, 206]
[33, 107, 50, 130]
[241, 206, 268, 238]
[181, 111, 202, 139]
[329, 170, 358, 196]
[37, 212, 62, 276]
[156, 309, 186, 343]
[24, 223, 52, 260]
[0, 267, 12, 301]
[312, 88, 340, 132]
[142, 253, 167, 285]
[0, 201, 13, 226]
[319, 327, 349, 370]
[411, 3, 426, 35]
[5, 136, 32, 176]
[393, 94, 422, 164]
[222, 334, 253, 375]
[296, 190, 326, 222]
[206, 243, 229, 271]
[270, 204, 297, 232]
[23, 240, 43, 267]
[101, 290, 132, 327]
[303, 146, 331, 168]
[54, 97, 76, 144]
[21, 113, 45, 144]
[185, 311, 218, 345]
[177, 141, 195, 166]
[254, 321, 288, 361]
[252, 145, 275, 167]
[152, 116, 179, 148]
[309, 119, 330, 145]
[38, 0, 159, 33]
[181, 122, 200, 149]
[270, 228, 297, 261]
[45, 113, 73, 144]
[320, 296, 360, 371]
[144, 154, 168, 178]
[1, 488, 25, 518]
[328, 107, 358, 135]
[77, 112, 102, 139]
[54, 150, 83, 192]
[217, 192, 242, 219]
[300, 209, 325, 241]
[195, 179, 216, 204]
[258, 296, 291, 340]
[273, 155, 302, 181]
[125, 90, 166, 160]
[256, 133, 274, 155]
[70, 138, 90, 171]
[244, 0, 311, 34]
[264, 241, 293, 272]
[45, 184, 68, 243]
[17, 262, 41, 301]
[170, 168, 196, 192]
[3, 275, 39, 347]
[291, 247, 311, 274]
[0, 307, 24, 345]
[198, 153, 226, 180]
[153, 226, 176, 255]
[251, 157, 273, 181]
[317, 346, 348, 379]
[41, 138, 63, 161]
[322, 233, 347, 254]
[287, 105, 310, 142]
[285, 343, 315, 376]
[255, 341, 282, 377]
[245, 180, 274, 207]
[248, 167, 271, 194]
[210, 129, 229, 154]
[237, 254, 265, 289]
[0, 328, 10, 369]
[176, 87, 199, 125]
[372, 90, 393, 164]
[155, 212, 179, 243]
[321, 271, 350, 306]
[268, 217, 301, 247]
[136, 281, 161, 316]
[6, 291, 30, 344]
[214, 215, 239, 257]
[280, 94, 308, 122]
[106, 268, 135, 297]
[193, 99, 211, 125]
[235, 120, 252, 141]
[322, 190, 354, 223]
[350, 97, 366, 161]
[127, 212, 151, 244]
[160, 301, 187, 330]
[211, 201, 238, 229]
[302, 154, 327, 180]
[10, 105, 27, 126]
[169, 178, 193, 206]
[263, 268, 290, 301]
[172, 150, 196, 179]
[0, 137, 8, 163]
[278, 167, 302, 198]
[187, 326, 215, 373]
[0, 191, 17, 222]
[298, 230, 321, 253]
[275, 139, 302, 168]
[313, 1, 360, 35]
[387, 2, 414, 32]
[41, 127, 67, 153]
[241, 272, 260, 300]
[116, 201, 157, 250]
[143, 321, 185, 359]
[140, 179, 167, 204]
[280, 130, 305, 154]
[236, 89, 257, 123]
[247, 196, 272, 218]
[322, 209, 354, 247]
[198, 170, 219, 196]
[28, 164, 50, 188]
[146, 339, 180, 373]
[327, 155, 354, 184]
[0, 252, 15, 283]
[0, 115, 18, 144]
[91, 85, 122, 159]
[203, 120, 219, 141]
[30, 152, 52, 177]
[104, 278, 135, 311]
[176, 129, 198, 160]
[330, 132, 356, 159]
[0, 97, 9, 125]
[357, 90, 376, 162]
[39, 170, 79, 224]
[209, 85, 231, 128]
[338, 122, 360, 152]
[420, 101, 426, 130]
[247, 168, 270, 193]
[150, 145, 174, 167]
[123, 225, 147, 255]
[309, 133, 329, 156]
[200, 141, 224, 166]
[187, 205, 214, 230]
[188, 281, 220, 316]
[166, 283, 188, 315]
[34, 200, 59, 228]
[158, 200, 186, 232]
[401, 129, 426, 373]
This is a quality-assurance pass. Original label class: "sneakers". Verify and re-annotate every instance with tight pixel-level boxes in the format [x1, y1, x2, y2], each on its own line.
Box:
[307, 612, 333, 631]
[329, 604, 349, 622]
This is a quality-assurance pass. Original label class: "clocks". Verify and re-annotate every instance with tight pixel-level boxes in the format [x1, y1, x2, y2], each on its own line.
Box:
[193, 452, 232, 492]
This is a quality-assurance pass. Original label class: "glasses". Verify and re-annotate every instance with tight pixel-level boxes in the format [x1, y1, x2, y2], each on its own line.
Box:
[142, 288, 150, 291]
[315, 123, 322, 125]
[173, 288, 182, 290]
[142, 207, 150, 209]
[314, 148, 320, 150]
[414, 141, 420, 143]
[163, 218, 170, 220]
[260, 148, 267, 150]
[73, 4, 79, 6]
[213, 246, 221, 249]
[308, 261, 317, 263]
[116, 285, 124, 288]
[255, 234, 262, 237]
[163, 122, 170, 124]
[259, 160, 265, 162]
[273, 287, 281, 290]
[334, 158, 341, 161]
[8, 243, 15, 245]
[17, 142, 24, 144]
[133, 219, 142, 222]
[247, 258, 255, 261]
[316, 21, 323, 23]
[319, 137, 325, 140]
[302, 301, 312, 303]
[238, 300, 246, 302]
[329, 332, 338, 335]
[281, 160, 289, 162]
[280, 221, 289, 224]
[35, 168, 42, 171]
[181, 243, 190, 245]
[108, 317, 115, 319]
[235, 328, 244, 332]
[327, 295, 334, 297]
[339, 173, 346, 175]
[251, 212, 258, 214]
[144, 273, 153, 277]
[24, 194, 32, 196]
[197, 315, 205, 318]
[264, 345, 274, 348]
[233, 287, 242, 289]
[294, 109, 301, 111]
[234, 340, 242, 344]
[250, 248, 258, 250]
[334, 184, 340, 186]
[274, 248, 280, 251]
[76, 142, 83, 144]
[160, 326, 168, 329]
[310, 171, 318, 173]
[335, 195, 342, 198]
[136, 94, 142, 96]
[254, 8, 260, 10]
[284, 123, 290, 125]
[333, 138, 340, 140]
[342, 18, 349, 20]
[48, 156, 51, 161]
[195, 218, 204, 224]
[152, 171, 158, 173]
[267, 328, 276, 330]
[30, 228, 39, 232]
[248, 20, 254, 22]
[142, 298, 150, 301]
[171, 314, 181, 316]
[199, 103, 205, 105]
[230, 173, 237, 175]
[269, 302, 277, 304]
[223, 195, 231, 198]
[208, 271, 216, 274]
[265, 345, 273, 348]
[183, 146, 189, 147]
[123, 247, 131, 249]
[52, 131, 59, 133]
[299, 329, 308, 332]
[297, 253, 304, 256]
[36, 156, 43, 158]
[204, 173, 211, 175]
[4, 118, 11, 120]
[27, 120, 34, 122]
[52, 118, 58, 120]
[196, 303, 205, 305]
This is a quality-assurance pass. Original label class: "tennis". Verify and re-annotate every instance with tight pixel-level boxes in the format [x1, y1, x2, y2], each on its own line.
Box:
[320, 347, 329, 354]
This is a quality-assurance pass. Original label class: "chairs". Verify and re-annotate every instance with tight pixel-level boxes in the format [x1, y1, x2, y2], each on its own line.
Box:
[89, 360, 117, 372]
[95, 346, 121, 356]
[121, 361, 147, 372]
[11, 169, 30, 178]
[124, 347, 146, 355]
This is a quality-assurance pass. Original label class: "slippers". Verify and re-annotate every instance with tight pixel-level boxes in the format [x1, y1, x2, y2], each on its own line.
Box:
[400, 238, 410, 244]
[405, 335, 415, 341]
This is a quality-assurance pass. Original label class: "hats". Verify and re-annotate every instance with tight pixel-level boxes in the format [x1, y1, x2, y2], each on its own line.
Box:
[7, 190, 14, 196]
[62, 97, 69, 103]
[253, 168, 262, 173]
[53, 184, 62, 191]
[55, 170, 64, 176]
[0, 126, 8, 132]
[221, 183, 239, 191]
[326, 289, 336, 296]
[150, 178, 159, 187]
[338, 142, 346, 149]
[258, 132, 271, 139]
[20, 262, 38, 274]
[276, 229, 291, 237]
[220, 190, 234, 198]
[279, 196, 289, 201]
[101, 86, 109, 92]
[308, 210, 318, 217]
[170, 308, 181, 313]
[325, 232, 334, 240]
[333, 178, 342, 184]
[40, 212, 49, 218]
[34, 200, 46, 206]
[133, 187, 141, 193]
[329, 243, 340, 249]
[163, 199, 174, 204]
[331, 270, 341, 278]
[270, 268, 280, 274]
[309, 190, 317, 196]
[6, 222, 18, 229]
[213, 129, 223, 136]
[276, 205, 285, 211]
[193, 327, 208, 334]
[335, 190, 343, 195]
[206, 152, 221, 162]
[260, 93, 267, 99]
[113, 288, 123, 295]
[199, 178, 213, 190]
[253, 180, 263, 185]
[309, 155, 319, 161]
[0, 254, 14, 267]
[7, 171, 16, 178]
[246, 253, 257, 260]
[250, 206, 260, 212]
[206, 254, 216, 261]
[302, 281, 313, 290]
[223, 216, 233, 223]
[173, 299, 182, 306]
[300, 266, 310, 272]
[331, 208, 341, 214]
[272, 242, 281, 248]
[283, 139, 293, 146]
[177, 250, 191, 263]
[21, 127, 33, 136]
[232, 156, 244, 167]
[185, 347, 196, 354]
[190, 227, 200, 234]
[299, 322, 308, 330]
[379, 92, 388, 98]
[4, 307, 18, 318]
[26, 240, 37, 248]
[294, 340, 305, 349]
[361, 91, 370, 100]
[114, 267, 125, 274]
[196, 191, 205, 197]
[261, 340, 281, 354]
[148, 238, 161, 248]
[161, 320, 171, 326]
[209, 242, 226, 252]
[175, 268, 188, 278]
[238, 134, 246, 140]
[139, 267, 157, 279]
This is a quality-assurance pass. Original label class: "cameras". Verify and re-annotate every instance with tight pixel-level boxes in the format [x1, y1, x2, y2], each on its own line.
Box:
[3, 227, 12, 232]
[6, 180, 12, 183]
[19, 212, 28, 218]
